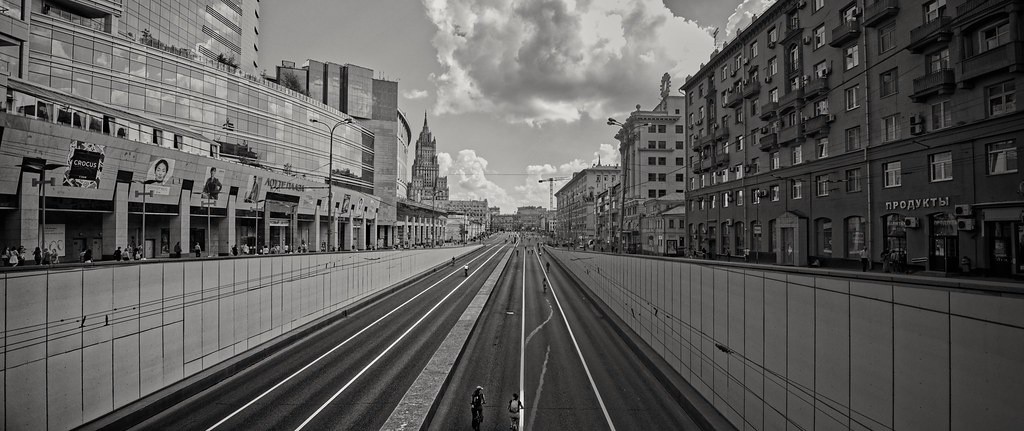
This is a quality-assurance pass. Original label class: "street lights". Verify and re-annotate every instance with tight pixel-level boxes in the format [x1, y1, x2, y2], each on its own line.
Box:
[310, 119, 355, 252]
[131, 180, 164, 259]
[424, 190, 444, 246]
[244, 199, 269, 255]
[193, 192, 226, 256]
[606, 118, 652, 254]
[15, 163, 69, 265]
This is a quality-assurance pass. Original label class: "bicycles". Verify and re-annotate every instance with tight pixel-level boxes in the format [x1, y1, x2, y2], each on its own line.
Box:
[470, 402, 523, 431]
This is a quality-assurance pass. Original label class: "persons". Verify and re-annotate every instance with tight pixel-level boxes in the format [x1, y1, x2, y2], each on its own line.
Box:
[201, 167, 222, 200]
[174, 242, 182, 258]
[541, 277, 548, 293]
[232, 240, 308, 256]
[463, 263, 469, 278]
[504, 234, 551, 273]
[470, 385, 486, 428]
[743, 246, 751, 263]
[244, 175, 259, 203]
[320, 243, 326, 253]
[880, 246, 908, 274]
[506, 392, 524, 431]
[342, 194, 350, 213]
[79, 248, 94, 264]
[151, 159, 169, 185]
[860, 246, 871, 272]
[356, 198, 364, 217]
[725, 246, 732, 261]
[194, 242, 201, 257]
[112, 243, 143, 261]
[451, 255, 456, 266]
[0, 246, 59, 269]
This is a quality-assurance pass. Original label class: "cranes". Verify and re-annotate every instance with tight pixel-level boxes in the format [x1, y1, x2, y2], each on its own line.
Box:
[538, 177, 572, 209]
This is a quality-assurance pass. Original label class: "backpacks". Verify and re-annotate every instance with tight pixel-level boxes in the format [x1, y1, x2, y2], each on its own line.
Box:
[510, 399, 518, 412]
[473, 390, 481, 407]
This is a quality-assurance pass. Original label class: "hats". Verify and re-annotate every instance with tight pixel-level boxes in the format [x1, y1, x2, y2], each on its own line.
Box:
[476, 386, 483, 390]
